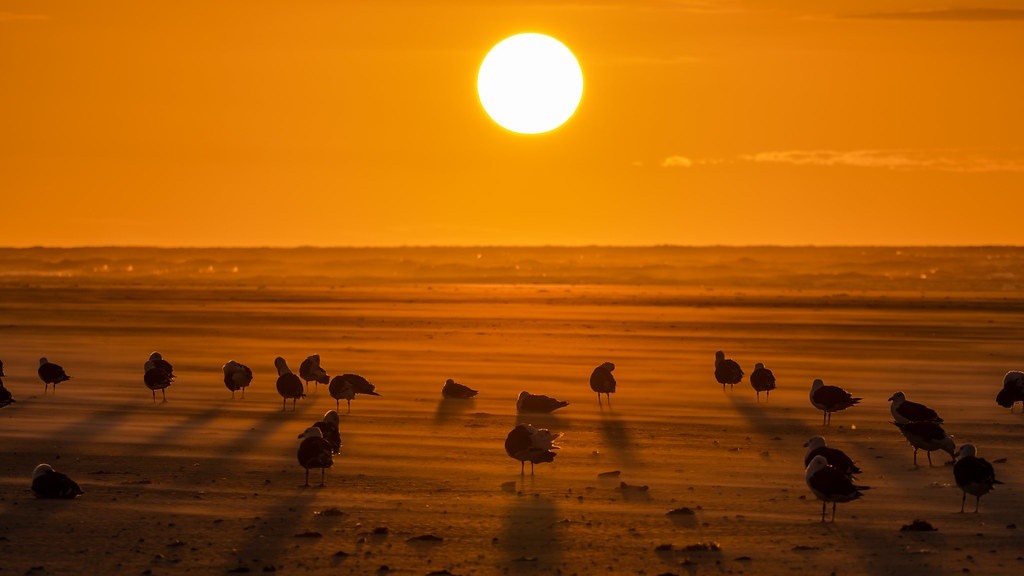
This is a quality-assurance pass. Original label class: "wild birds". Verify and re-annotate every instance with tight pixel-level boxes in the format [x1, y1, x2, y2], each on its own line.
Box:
[996, 370, 1024, 409]
[809, 378, 863, 426]
[589, 361, 617, 408]
[222, 360, 252, 399]
[802, 436, 873, 524]
[887, 390, 1007, 515]
[715, 350, 778, 402]
[38, 357, 72, 397]
[515, 390, 571, 415]
[275, 353, 383, 415]
[0, 360, 27, 412]
[505, 421, 565, 478]
[296, 410, 343, 489]
[441, 379, 480, 400]
[143, 351, 178, 405]
[31, 463, 85, 500]
